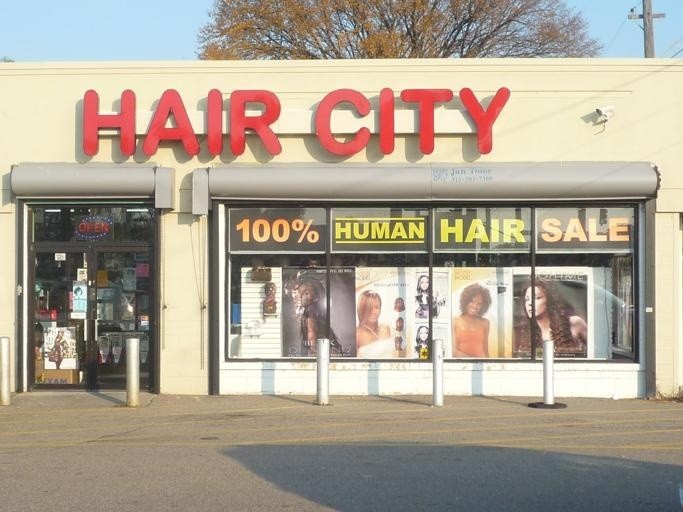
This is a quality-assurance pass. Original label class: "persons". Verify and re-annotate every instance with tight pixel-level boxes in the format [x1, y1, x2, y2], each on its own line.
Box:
[294, 278, 343, 359]
[514, 281, 586, 356]
[395, 317, 405, 331]
[449, 282, 492, 357]
[414, 275, 440, 319]
[393, 336, 403, 351]
[73, 286, 83, 298]
[354, 290, 392, 356]
[394, 297, 405, 313]
[106, 261, 123, 288]
[412, 325, 433, 360]
[52, 329, 67, 369]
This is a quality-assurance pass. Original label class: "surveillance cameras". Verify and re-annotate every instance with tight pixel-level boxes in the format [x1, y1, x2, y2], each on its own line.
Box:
[595, 104, 613, 117]
